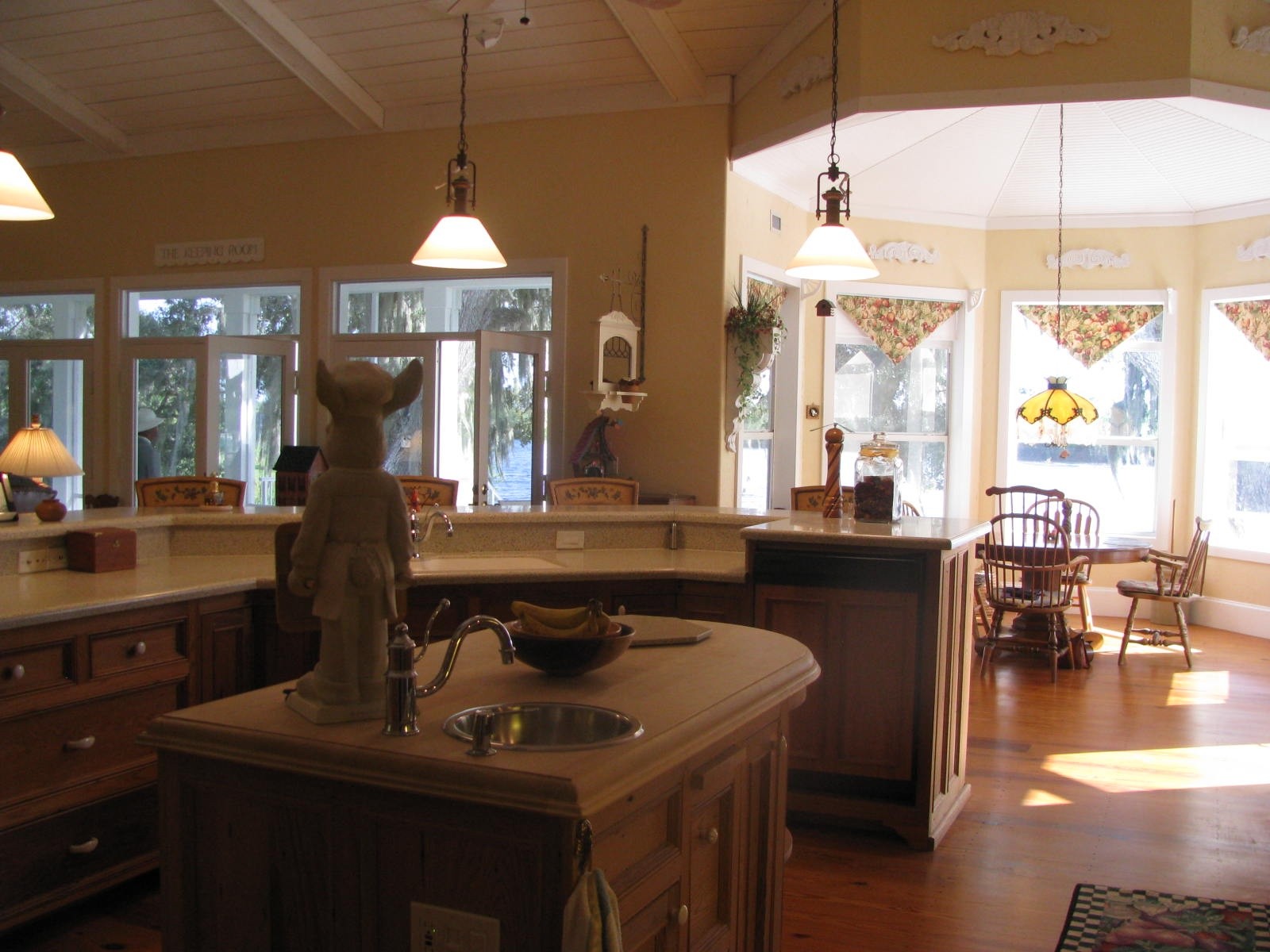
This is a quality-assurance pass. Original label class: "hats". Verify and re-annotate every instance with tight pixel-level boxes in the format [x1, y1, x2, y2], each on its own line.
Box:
[138, 408, 165, 433]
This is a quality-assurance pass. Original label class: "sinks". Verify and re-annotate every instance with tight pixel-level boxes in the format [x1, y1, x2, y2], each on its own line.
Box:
[441, 702, 644, 752]
[407, 556, 563, 571]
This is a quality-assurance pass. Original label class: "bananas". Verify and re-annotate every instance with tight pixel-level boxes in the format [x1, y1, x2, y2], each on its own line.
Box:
[510, 596, 614, 639]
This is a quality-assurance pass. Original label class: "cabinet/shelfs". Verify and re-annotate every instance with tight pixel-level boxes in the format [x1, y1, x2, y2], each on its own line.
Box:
[135, 610, 820, 952]
[1, 547, 976, 923]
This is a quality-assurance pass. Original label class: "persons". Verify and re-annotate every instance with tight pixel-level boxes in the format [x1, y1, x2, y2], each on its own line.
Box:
[138, 407, 165, 478]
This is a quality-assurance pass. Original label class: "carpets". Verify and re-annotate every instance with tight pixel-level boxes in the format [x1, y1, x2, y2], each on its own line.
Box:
[1056, 884, 1269, 952]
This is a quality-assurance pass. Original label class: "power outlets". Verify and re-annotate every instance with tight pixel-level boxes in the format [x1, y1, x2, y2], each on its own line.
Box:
[408, 902, 500, 951]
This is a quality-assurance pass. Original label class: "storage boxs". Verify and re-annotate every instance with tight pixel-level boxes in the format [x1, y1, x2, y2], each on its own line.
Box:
[67, 526, 137, 572]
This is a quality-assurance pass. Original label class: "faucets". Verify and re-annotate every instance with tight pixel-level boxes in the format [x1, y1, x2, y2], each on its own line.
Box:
[412, 511, 455, 545]
[414, 614, 517, 698]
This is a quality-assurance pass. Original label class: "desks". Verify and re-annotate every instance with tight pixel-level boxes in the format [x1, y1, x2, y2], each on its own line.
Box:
[975, 529, 1150, 650]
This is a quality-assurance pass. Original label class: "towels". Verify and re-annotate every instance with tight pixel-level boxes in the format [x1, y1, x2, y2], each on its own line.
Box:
[561, 867, 624, 952]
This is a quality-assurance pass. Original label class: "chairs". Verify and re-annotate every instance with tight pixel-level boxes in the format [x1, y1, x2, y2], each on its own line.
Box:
[979, 484, 1211, 682]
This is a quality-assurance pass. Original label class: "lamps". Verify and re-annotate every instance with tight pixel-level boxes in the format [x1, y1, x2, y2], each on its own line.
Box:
[787, 0, 880, 281]
[1, 1, 54, 221]
[1016, 103, 1098, 426]
[0, 414, 84, 521]
[411, 14, 507, 269]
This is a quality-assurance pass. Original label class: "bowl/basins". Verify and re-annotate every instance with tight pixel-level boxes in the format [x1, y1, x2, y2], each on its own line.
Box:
[509, 621, 635, 677]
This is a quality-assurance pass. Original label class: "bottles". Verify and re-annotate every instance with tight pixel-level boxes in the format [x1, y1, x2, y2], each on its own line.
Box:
[855, 433, 903, 524]
[35, 499, 67, 522]
